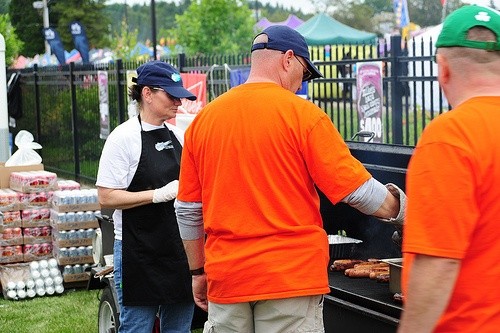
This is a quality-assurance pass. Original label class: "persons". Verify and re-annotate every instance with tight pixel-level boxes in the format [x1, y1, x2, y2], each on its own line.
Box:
[96, 61, 195, 333]
[172, 25, 406, 333]
[398, 4, 500, 333]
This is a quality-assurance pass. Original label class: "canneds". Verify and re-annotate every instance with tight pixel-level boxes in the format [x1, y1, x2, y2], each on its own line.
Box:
[52, 189, 100, 273]
[6, 259, 64, 300]
[0, 170, 80, 257]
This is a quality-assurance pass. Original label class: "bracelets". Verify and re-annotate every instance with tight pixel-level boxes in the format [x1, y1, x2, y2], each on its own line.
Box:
[188, 266, 204, 276]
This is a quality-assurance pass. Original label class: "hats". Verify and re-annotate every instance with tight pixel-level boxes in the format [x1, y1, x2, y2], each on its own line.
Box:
[434, 4, 500, 64]
[251, 24, 323, 82]
[136, 61, 197, 101]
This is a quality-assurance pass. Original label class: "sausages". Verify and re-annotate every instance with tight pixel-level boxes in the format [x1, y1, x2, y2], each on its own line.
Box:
[331, 259, 389, 281]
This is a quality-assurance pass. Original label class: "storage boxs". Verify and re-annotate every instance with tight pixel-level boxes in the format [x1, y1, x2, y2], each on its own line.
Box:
[0, 162, 101, 282]
[381, 257, 403, 295]
[325, 234, 364, 259]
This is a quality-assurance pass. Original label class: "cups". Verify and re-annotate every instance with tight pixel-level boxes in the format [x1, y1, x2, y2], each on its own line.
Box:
[104, 254, 113, 266]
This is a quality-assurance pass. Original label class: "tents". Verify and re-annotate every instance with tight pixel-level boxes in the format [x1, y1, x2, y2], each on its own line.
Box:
[10, 41, 184, 69]
[408, 22, 452, 113]
[256, 13, 377, 99]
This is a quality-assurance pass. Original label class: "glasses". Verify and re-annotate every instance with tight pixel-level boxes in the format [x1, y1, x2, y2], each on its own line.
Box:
[281, 52, 312, 81]
[153, 87, 180, 100]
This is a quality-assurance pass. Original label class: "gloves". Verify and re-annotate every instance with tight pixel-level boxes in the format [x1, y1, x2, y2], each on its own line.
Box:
[152, 180, 179, 204]
